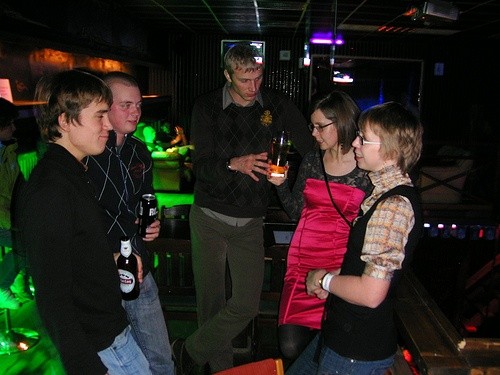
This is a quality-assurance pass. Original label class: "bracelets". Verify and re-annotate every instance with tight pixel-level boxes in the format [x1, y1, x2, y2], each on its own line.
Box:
[322, 273, 334, 293]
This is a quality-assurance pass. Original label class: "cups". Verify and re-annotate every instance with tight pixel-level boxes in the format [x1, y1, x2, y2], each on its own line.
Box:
[268, 137, 289, 178]
[0, 309, 14, 346]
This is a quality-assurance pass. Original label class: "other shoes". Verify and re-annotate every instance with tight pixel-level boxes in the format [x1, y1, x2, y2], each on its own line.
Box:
[171, 339, 192, 375]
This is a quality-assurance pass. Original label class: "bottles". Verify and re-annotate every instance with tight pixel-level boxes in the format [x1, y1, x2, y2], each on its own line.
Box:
[117, 235, 139, 301]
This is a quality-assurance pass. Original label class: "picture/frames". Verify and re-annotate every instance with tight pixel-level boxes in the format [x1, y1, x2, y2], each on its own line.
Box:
[220, 39, 265, 69]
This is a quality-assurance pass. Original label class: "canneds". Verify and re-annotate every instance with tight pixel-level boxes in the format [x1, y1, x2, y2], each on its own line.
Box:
[138, 194, 158, 238]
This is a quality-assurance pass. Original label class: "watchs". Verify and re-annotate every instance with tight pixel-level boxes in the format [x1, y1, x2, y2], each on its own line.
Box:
[227, 159, 231, 169]
[318, 272, 329, 292]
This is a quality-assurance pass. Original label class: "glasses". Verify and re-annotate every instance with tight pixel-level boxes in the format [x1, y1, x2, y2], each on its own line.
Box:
[355, 130, 381, 146]
[309, 122, 334, 133]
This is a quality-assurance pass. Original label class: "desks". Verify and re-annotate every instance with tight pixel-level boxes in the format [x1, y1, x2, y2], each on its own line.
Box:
[142, 219, 195, 295]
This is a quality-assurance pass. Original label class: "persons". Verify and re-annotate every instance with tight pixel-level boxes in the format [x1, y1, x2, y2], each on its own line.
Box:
[306, 100, 423, 375]
[275, 92, 372, 374]
[169, 44, 304, 375]
[82, 70, 175, 374]
[12, 65, 153, 375]
